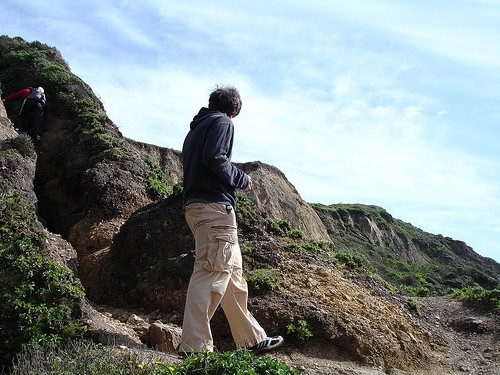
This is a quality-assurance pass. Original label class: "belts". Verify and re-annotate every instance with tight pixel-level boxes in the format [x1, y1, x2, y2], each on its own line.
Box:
[185, 199, 208, 207]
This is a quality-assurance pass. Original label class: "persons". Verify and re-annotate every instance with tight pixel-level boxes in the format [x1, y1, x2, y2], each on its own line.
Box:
[178, 84, 285, 361]
[2, 82, 47, 143]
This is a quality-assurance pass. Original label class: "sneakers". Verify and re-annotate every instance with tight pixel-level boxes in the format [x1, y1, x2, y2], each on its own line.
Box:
[246, 335, 283, 351]
